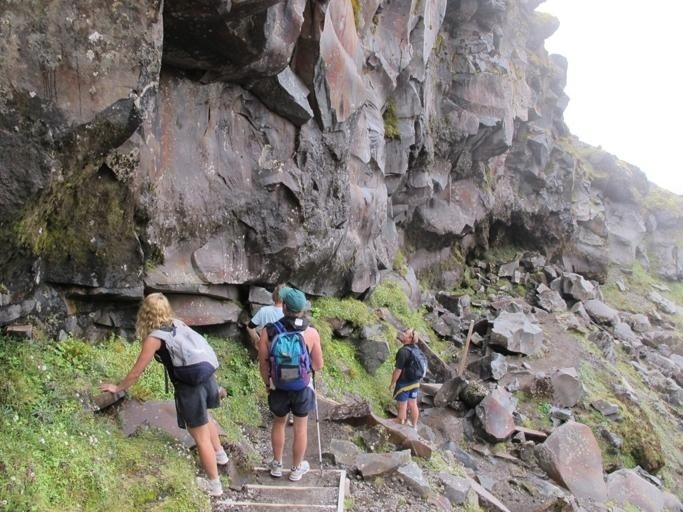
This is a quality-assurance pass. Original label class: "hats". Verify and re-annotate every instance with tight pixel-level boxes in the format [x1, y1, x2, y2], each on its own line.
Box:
[279, 288, 306, 312]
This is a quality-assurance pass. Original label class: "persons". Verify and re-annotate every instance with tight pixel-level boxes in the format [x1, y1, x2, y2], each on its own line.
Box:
[389, 329, 419, 432]
[259, 286, 323, 481]
[97, 292, 228, 496]
[246, 284, 294, 424]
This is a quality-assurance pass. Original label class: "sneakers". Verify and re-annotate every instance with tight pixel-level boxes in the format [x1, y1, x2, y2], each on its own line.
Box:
[267, 459, 282, 476]
[289, 461, 309, 481]
[213, 447, 228, 464]
[196, 477, 222, 496]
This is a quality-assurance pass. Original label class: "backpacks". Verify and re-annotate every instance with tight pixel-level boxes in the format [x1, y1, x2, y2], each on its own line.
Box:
[270, 321, 311, 391]
[147, 317, 218, 385]
[403, 344, 427, 380]
